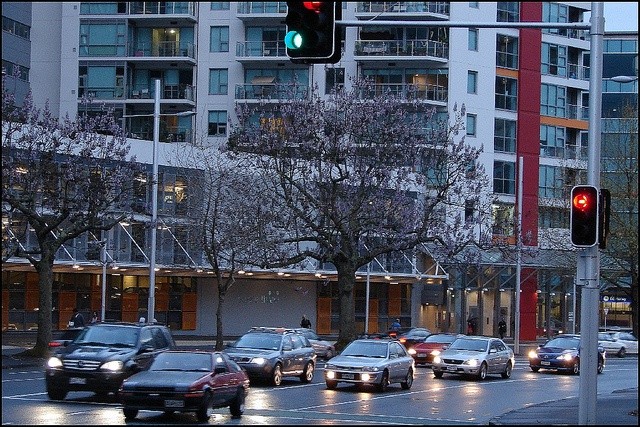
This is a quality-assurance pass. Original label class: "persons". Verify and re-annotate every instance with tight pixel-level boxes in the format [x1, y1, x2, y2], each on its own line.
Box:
[388, 318, 401, 330]
[299, 314, 312, 329]
[67, 306, 85, 327]
[497, 315, 507, 337]
[87, 311, 98, 325]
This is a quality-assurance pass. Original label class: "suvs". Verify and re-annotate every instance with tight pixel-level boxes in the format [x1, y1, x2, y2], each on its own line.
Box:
[598, 332, 628, 358]
[46, 319, 177, 402]
[226, 327, 317, 386]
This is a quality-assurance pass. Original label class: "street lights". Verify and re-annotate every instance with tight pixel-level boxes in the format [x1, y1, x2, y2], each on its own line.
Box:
[122, 76, 197, 321]
[583, 76, 637, 320]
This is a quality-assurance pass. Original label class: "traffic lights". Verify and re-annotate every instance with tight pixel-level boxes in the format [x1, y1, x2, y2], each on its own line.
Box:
[569, 185, 599, 248]
[285, 0, 342, 64]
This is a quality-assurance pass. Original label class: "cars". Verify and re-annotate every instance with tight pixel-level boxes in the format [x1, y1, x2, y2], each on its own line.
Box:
[407, 334, 465, 366]
[432, 336, 515, 380]
[324, 340, 414, 392]
[612, 332, 638, 354]
[302, 328, 335, 359]
[117, 348, 251, 422]
[396, 327, 432, 348]
[529, 336, 603, 374]
